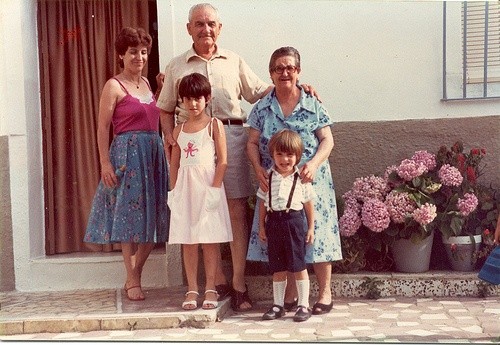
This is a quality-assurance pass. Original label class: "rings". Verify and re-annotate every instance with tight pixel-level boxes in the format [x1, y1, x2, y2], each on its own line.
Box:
[260, 182, 263, 185]
[105, 176, 108, 179]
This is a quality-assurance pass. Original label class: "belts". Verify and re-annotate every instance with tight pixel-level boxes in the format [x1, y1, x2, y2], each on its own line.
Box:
[220, 119, 242, 125]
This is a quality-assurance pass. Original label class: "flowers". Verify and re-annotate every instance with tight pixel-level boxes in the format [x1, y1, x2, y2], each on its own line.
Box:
[337, 141, 500, 258]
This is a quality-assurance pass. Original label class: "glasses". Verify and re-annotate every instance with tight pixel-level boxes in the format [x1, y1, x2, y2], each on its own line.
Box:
[273, 65, 297, 73]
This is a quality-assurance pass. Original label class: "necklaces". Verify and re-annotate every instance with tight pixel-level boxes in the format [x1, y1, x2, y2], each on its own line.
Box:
[123, 72, 141, 88]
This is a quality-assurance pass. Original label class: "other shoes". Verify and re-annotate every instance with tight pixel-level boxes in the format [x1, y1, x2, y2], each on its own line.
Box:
[294, 306, 311, 321]
[263, 305, 284, 319]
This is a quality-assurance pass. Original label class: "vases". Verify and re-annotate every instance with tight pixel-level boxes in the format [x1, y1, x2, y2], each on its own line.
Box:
[392, 230, 434, 273]
[443, 235, 481, 272]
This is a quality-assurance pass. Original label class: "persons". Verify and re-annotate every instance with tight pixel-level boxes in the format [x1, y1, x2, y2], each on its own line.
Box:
[245, 47, 344, 315]
[167, 72, 227, 311]
[83, 28, 170, 300]
[155, 3, 318, 312]
[255, 129, 317, 322]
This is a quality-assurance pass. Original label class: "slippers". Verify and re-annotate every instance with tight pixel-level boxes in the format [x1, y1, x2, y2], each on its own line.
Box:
[312, 301, 333, 314]
[284, 298, 298, 311]
[124, 283, 145, 301]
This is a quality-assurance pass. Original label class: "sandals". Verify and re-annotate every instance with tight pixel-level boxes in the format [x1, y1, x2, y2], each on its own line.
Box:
[202, 290, 220, 309]
[182, 291, 199, 309]
[232, 284, 253, 311]
[216, 283, 231, 300]
